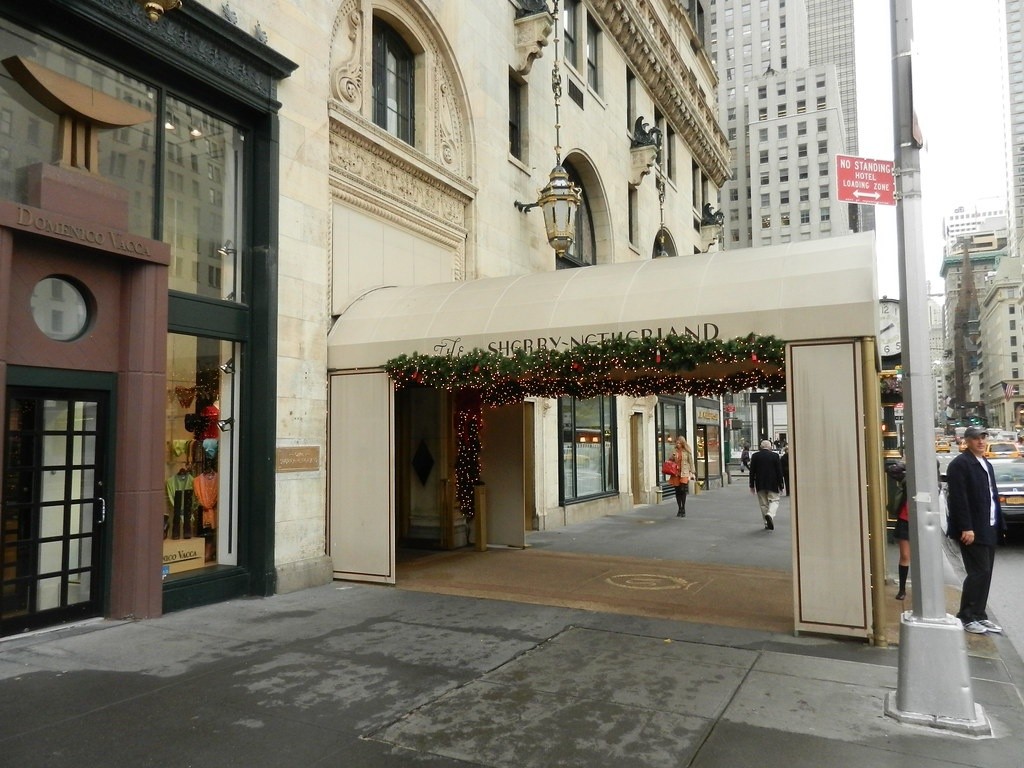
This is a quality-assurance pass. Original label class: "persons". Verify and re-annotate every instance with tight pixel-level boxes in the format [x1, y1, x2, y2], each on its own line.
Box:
[947, 424, 1007, 634]
[202, 399, 220, 440]
[887, 450, 942, 600]
[780, 446, 790, 497]
[741, 437, 788, 472]
[667, 436, 695, 517]
[749, 440, 784, 530]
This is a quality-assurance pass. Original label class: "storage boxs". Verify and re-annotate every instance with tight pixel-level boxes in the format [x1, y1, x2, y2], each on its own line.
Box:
[162, 536, 204, 574]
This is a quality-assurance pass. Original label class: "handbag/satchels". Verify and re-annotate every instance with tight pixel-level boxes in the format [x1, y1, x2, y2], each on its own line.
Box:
[886, 476, 906, 515]
[662, 460, 678, 476]
[688, 474, 696, 497]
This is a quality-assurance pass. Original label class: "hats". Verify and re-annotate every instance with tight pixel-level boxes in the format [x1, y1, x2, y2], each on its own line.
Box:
[964, 425, 989, 439]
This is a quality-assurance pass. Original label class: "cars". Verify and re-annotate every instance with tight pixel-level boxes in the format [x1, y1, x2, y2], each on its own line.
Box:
[937, 427, 968, 446]
[938, 453, 1024, 538]
[935, 441, 952, 453]
[986, 428, 1018, 442]
[983, 441, 1022, 459]
[958, 439, 968, 452]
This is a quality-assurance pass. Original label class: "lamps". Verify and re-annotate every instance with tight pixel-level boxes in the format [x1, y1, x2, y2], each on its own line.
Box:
[217, 417, 233, 432]
[219, 358, 233, 375]
[515, 0, 584, 261]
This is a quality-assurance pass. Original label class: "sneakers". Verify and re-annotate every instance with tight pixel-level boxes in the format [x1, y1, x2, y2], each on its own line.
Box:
[963, 620, 1003, 633]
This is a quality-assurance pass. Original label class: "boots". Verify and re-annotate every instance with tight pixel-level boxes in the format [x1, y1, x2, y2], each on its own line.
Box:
[896, 565, 909, 599]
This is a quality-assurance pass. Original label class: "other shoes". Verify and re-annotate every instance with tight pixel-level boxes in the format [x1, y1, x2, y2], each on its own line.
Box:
[764, 513, 774, 530]
[677, 508, 685, 517]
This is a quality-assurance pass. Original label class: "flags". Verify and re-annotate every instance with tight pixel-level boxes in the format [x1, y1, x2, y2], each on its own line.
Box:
[1002, 381, 1014, 401]
